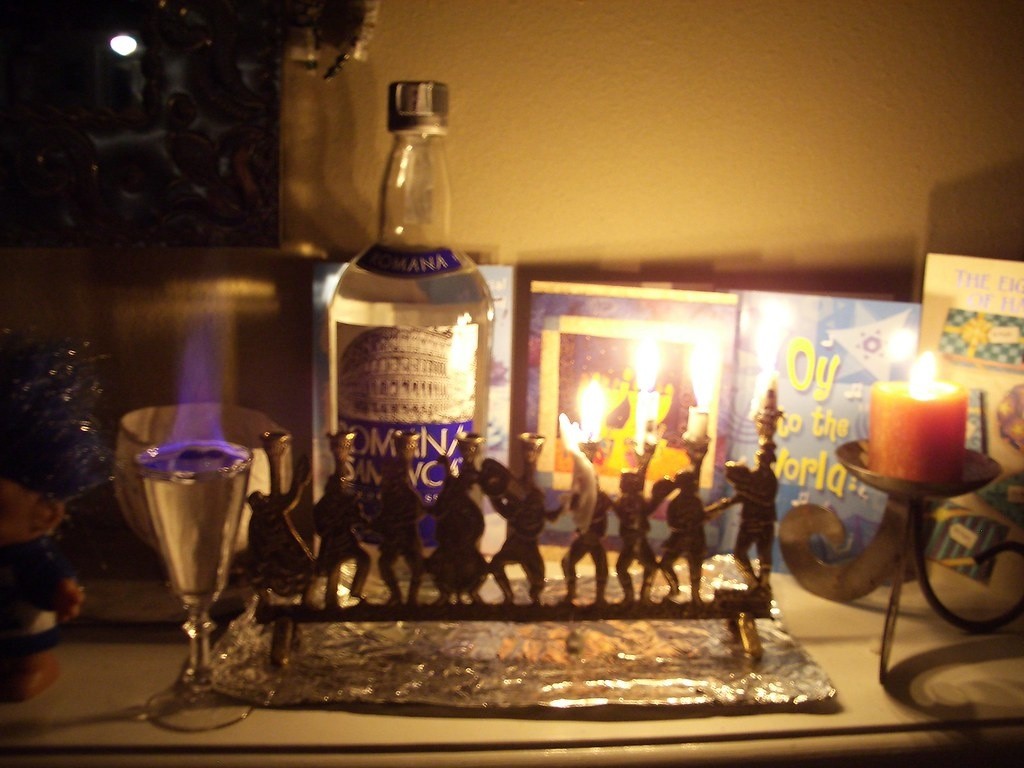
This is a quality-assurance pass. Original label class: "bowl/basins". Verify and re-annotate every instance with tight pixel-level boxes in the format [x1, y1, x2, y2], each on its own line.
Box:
[115, 403, 289, 557]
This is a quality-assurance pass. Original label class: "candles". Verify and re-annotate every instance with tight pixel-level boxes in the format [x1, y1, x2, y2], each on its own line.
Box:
[868, 352, 970, 483]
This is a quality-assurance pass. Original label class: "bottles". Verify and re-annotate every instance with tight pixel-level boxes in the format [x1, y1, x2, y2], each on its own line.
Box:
[324, 81, 495, 572]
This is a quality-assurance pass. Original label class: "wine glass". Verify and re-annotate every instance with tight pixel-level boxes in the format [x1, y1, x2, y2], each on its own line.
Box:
[130, 437, 252, 734]
